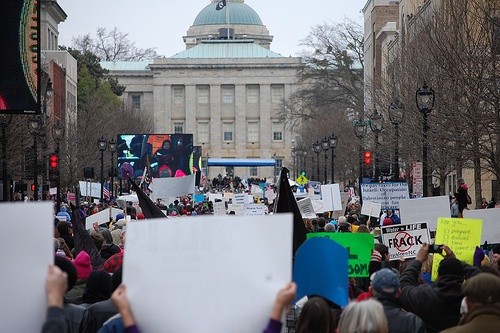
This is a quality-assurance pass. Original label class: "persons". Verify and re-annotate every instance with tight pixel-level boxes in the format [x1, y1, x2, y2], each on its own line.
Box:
[453, 178, 468, 215]
[247, 177, 500, 333]
[13, 173, 243, 333]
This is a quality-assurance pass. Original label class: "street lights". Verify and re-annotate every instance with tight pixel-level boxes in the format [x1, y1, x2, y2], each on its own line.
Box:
[108, 137, 118, 207]
[290, 137, 329, 183]
[51, 119, 65, 210]
[369, 103, 385, 183]
[26, 115, 42, 200]
[387, 96, 406, 182]
[96, 135, 108, 210]
[328, 132, 340, 185]
[415, 80, 436, 197]
[353, 115, 368, 204]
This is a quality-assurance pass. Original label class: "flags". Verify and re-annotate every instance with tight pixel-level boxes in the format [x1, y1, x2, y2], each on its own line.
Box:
[275, 166, 307, 259]
[127, 175, 170, 218]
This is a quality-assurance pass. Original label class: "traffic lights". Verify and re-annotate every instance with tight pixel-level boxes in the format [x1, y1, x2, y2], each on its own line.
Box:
[49, 152, 59, 182]
[363, 148, 372, 170]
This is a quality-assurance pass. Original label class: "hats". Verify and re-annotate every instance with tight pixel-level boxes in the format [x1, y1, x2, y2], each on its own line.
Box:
[370, 268, 402, 297]
[72, 251, 93, 279]
[370, 227, 381, 238]
[116, 213, 124, 220]
[384, 219, 393, 226]
[324, 223, 335, 232]
[83, 270, 111, 299]
